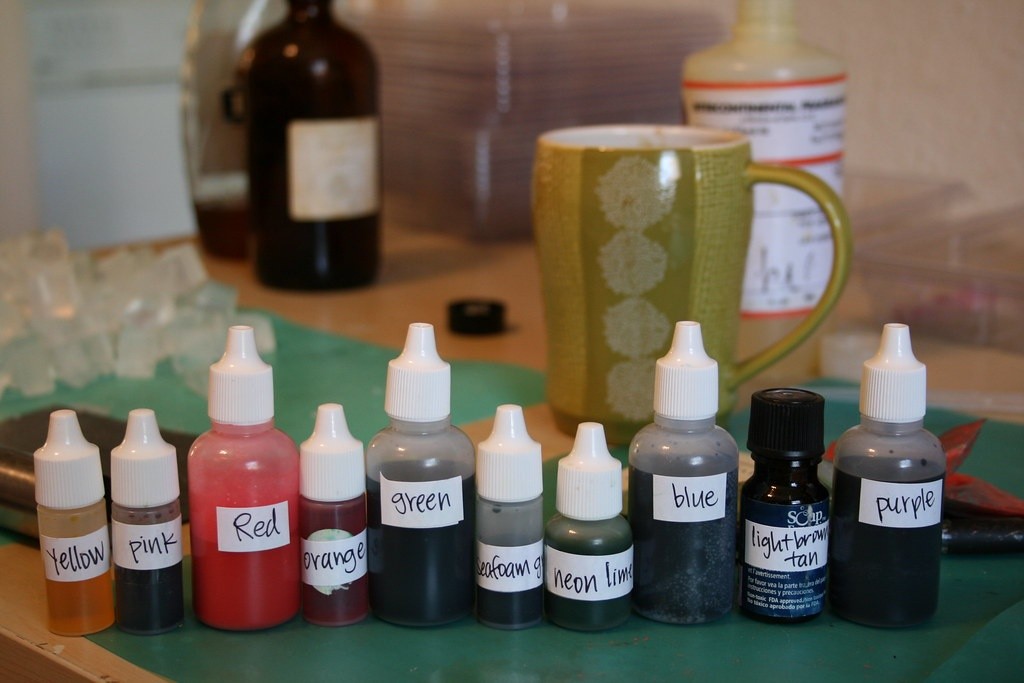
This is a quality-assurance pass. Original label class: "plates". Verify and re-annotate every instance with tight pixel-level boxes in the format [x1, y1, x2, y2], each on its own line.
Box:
[373, 0, 730, 234]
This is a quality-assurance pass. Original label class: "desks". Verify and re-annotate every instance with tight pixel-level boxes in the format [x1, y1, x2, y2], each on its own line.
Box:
[0, 217, 1024, 683]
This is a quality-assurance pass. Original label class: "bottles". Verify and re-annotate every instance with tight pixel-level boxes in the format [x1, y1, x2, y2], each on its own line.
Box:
[33, 410, 116, 636]
[545, 422, 633, 630]
[241, 0, 378, 287]
[367, 323, 472, 628]
[681, 0, 847, 387]
[187, 326, 299, 630]
[628, 321, 739, 624]
[299, 403, 368, 626]
[740, 390, 831, 626]
[110, 409, 184, 635]
[835, 322, 945, 628]
[474, 404, 543, 629]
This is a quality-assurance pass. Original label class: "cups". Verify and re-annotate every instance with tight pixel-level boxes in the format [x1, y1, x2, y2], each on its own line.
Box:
[531, 125, 853, 443]
